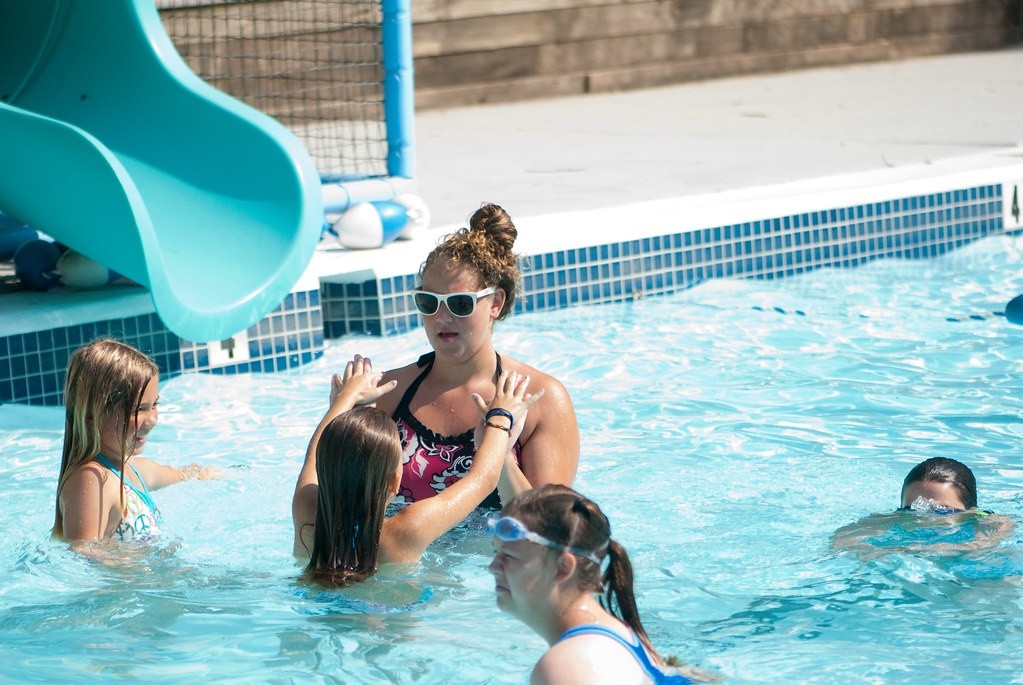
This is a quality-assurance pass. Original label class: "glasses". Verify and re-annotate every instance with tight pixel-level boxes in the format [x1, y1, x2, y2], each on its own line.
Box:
[899, 505, 954, 517]
[409, 285, 497, 318]
[484, 517, 525, 543]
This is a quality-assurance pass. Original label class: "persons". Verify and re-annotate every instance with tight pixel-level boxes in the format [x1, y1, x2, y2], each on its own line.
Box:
[329, 203, 579, 510]
[52, 339, 181, 538]
[290, 354, 546, 572]
[900, 457, 977, 510]
[491, 482, 664, 684]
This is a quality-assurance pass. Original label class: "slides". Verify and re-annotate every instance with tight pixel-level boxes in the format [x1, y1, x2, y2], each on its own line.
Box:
[4, 0, 328, 344]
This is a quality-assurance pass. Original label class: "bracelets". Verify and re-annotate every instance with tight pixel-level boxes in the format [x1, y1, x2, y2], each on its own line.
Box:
[485, 421, 512, 436]
[485, 408, 514, 430]
